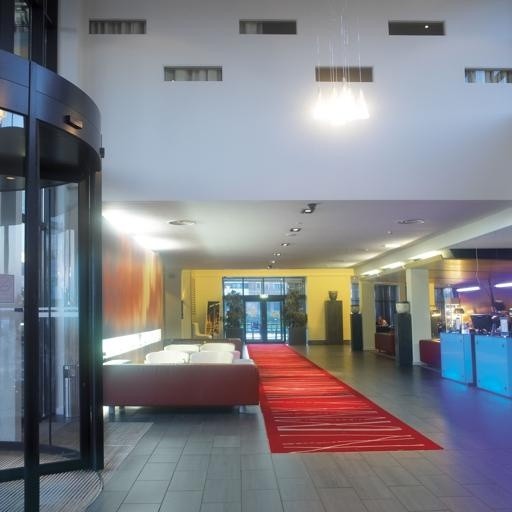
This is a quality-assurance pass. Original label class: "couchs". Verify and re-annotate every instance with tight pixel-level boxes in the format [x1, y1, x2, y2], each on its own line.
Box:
[102, 359, 261, 418]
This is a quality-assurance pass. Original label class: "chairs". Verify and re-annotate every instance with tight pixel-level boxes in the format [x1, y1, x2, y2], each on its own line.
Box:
[145, 343, 235, 364]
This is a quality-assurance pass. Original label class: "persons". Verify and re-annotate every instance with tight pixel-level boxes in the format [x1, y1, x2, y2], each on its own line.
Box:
[377, 315, 389, 326]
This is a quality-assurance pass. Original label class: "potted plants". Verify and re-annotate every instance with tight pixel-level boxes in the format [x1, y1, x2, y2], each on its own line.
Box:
[282, 288, 307, 345]
[222, 290, 245, 345]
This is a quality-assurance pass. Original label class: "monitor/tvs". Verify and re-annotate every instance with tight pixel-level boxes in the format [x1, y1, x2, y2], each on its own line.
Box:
[470, 313, 492, 330]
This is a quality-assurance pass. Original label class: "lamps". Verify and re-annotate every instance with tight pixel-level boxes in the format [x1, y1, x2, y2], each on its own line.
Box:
[310, 6, 369, 121]
